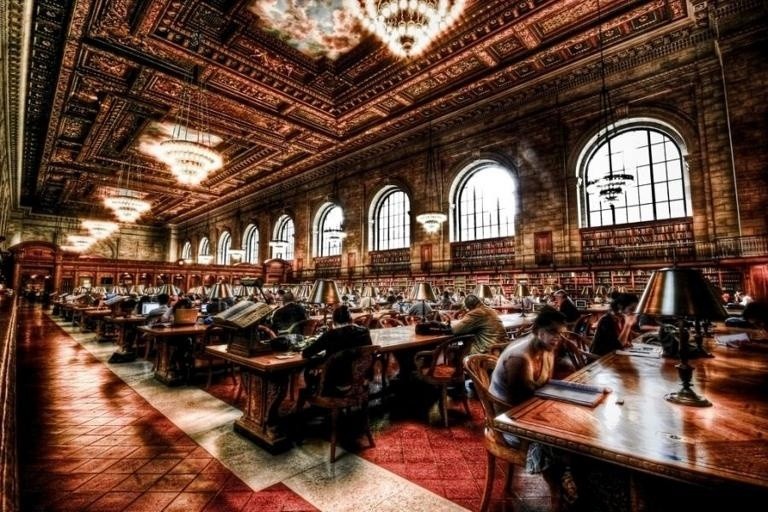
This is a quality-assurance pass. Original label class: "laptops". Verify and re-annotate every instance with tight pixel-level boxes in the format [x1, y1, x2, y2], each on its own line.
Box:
[172, 309, 198, 324]
[143, 302, 158, 316]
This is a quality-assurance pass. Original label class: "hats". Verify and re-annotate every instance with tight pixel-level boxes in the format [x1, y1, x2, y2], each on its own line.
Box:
[531, 304, 566, 336]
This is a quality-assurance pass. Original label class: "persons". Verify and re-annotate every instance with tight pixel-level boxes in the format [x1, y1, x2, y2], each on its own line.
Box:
[1, 284, 767, 511]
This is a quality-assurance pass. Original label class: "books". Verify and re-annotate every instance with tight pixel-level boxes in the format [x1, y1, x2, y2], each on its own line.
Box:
[310, 219, 696, 284]
